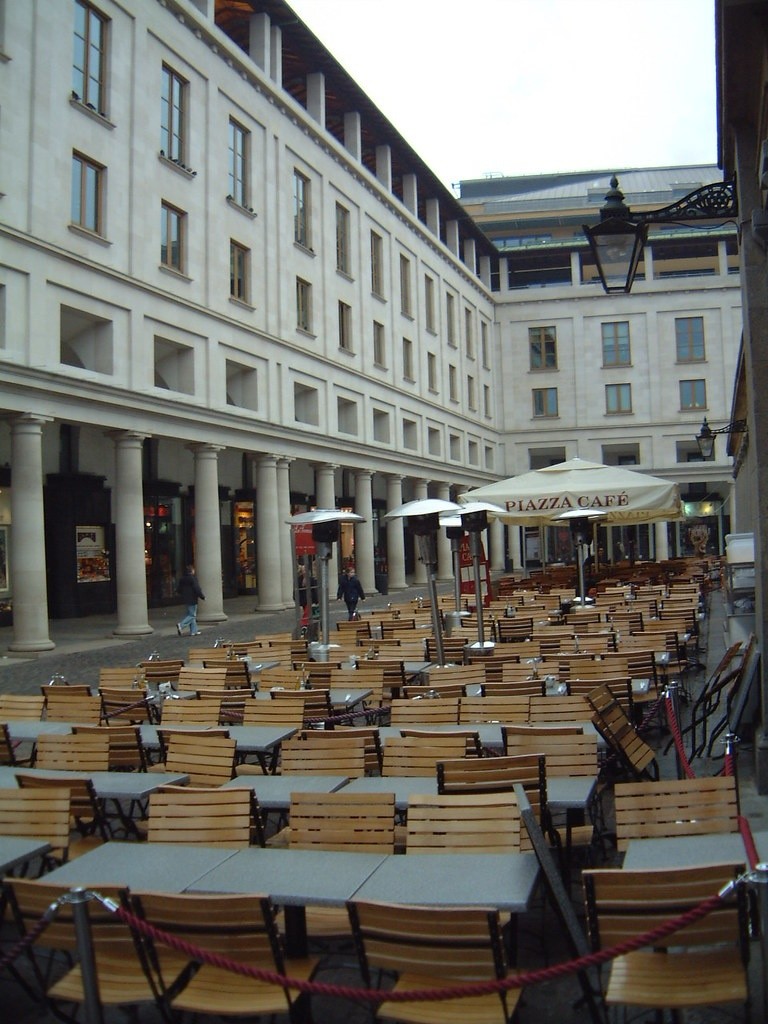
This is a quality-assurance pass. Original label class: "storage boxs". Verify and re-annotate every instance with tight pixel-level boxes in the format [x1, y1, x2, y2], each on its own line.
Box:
[725, 533, 754, 563]
[730, 564, 755, 588]
[726, 613, 755, 648]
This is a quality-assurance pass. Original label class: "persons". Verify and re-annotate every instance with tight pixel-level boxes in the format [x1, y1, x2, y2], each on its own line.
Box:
[175, 565, 208, 636]
[336, 567, 366, 621]
[293, 566, 318, 612]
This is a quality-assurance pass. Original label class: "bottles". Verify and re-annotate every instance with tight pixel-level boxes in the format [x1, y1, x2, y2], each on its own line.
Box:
[226, 649, 239, 660]
[131, 676, 148, 689]
[293, 675, 311, 691]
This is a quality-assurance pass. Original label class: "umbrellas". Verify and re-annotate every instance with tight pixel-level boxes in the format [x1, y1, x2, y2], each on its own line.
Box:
[456, 458, 686, 609]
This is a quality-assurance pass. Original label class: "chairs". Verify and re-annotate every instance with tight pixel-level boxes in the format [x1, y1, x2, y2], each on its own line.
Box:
[0, 553, 768, 1024]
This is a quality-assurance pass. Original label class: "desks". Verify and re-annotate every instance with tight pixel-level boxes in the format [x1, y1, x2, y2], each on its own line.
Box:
[0, 568, 768, 1024]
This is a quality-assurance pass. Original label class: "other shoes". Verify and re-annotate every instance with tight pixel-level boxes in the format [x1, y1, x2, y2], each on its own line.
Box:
[190, 632, 201, 636]
[177, 623, 182, 635]
